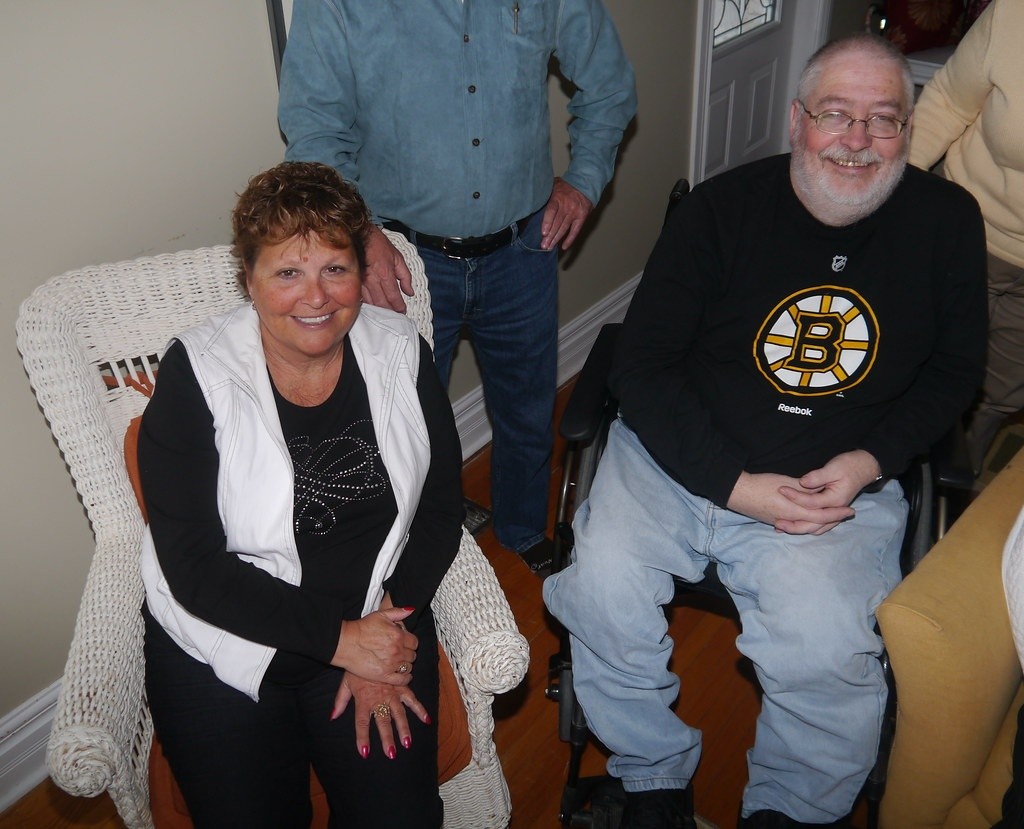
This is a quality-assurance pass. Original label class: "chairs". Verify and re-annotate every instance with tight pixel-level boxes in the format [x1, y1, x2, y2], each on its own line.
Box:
[17, 227, 530, 829]
[876, 446, 1024, 828]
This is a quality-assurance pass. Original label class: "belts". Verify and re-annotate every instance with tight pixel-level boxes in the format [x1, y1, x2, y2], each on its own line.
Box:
[380, 217, 533, 261]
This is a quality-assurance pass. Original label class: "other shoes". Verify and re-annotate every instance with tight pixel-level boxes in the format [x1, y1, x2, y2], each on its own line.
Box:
[738, 801, 801, 829]
[625, 781, 696, 829]
[517, 538, 570, 580]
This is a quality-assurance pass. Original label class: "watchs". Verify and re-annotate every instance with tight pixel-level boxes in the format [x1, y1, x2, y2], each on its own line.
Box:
[863, 474, 883, 492]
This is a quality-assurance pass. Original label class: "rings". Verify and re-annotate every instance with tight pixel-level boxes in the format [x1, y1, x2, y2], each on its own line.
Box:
[398, 661, 408, 674]
[372, 703, 392, 720]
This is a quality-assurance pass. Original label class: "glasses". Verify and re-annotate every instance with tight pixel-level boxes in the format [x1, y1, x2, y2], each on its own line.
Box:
[802, 101, 910, 140]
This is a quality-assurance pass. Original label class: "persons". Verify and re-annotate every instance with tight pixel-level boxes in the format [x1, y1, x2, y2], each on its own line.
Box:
[544, 30, 989, 828]
[907, 0, 1024, 539]
[277, 1, 637, 575]
[139, 161, 466, 829]
